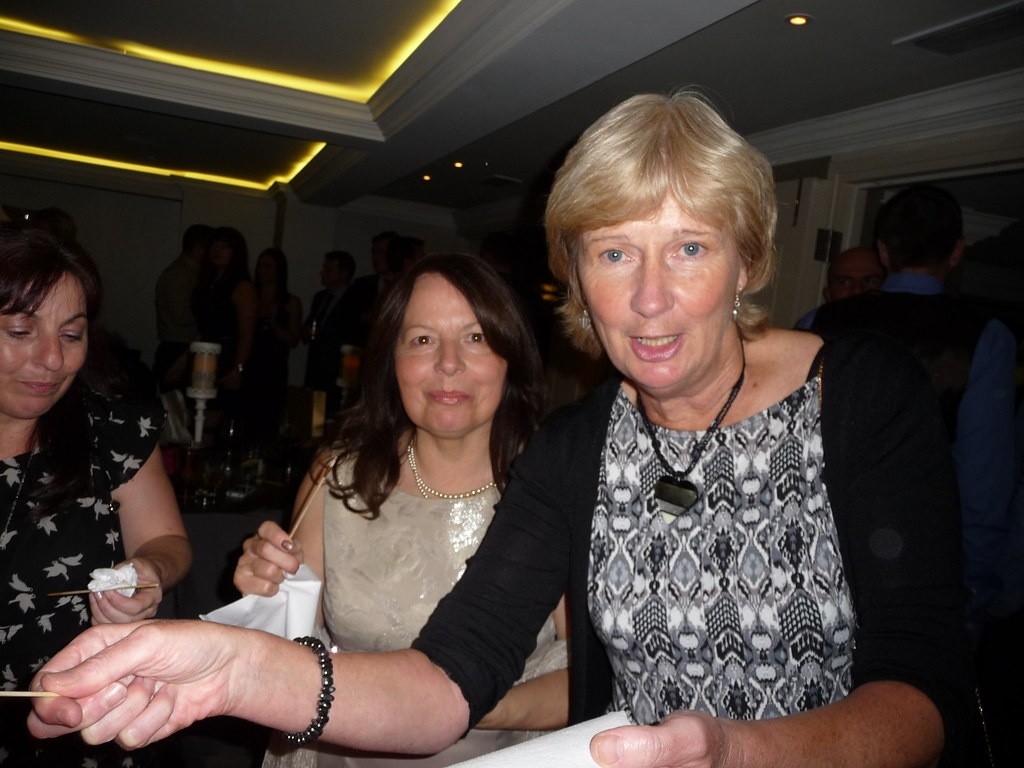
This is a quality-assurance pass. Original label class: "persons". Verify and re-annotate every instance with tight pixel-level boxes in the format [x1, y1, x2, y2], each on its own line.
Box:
[0, 93, 1024, 768]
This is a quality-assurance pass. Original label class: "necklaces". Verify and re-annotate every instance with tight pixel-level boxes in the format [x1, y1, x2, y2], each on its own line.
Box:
[631, 325, 747, 507]
[0, 447, 35, 530]
[408, 437, 495, 499]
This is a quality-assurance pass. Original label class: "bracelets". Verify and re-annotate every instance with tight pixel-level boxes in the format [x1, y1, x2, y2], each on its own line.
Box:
[284, 636, 334, 745]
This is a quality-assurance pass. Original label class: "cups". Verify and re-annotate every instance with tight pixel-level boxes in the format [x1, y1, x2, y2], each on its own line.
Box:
[308, 319, 320, 340]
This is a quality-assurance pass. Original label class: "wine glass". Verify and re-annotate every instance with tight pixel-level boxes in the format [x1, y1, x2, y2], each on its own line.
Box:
[220, 416, 242, 466]
